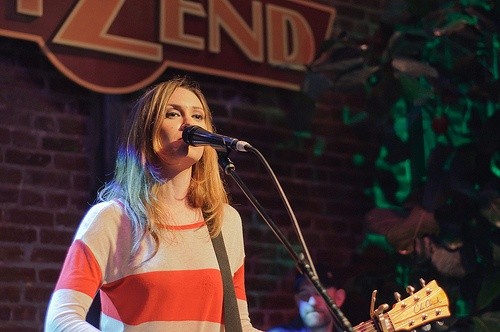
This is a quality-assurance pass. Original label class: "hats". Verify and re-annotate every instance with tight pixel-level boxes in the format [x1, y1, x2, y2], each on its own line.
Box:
[292, 261, 344, 293]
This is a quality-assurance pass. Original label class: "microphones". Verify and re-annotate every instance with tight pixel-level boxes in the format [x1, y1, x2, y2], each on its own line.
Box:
[182, 125, 251, 152]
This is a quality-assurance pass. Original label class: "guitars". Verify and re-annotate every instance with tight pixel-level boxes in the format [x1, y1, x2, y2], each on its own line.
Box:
[350, 276, 451, 332]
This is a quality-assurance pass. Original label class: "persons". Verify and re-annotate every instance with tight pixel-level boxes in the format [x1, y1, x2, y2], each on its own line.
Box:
[267, 267, 346, 332]
[43, 80, 265, 332]
[413, 221, 481, 332]
